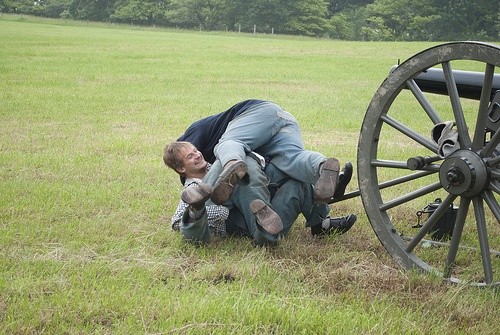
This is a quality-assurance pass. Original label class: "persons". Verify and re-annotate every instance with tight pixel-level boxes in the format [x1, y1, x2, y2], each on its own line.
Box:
[162, 137, 358, 241]
[177, 150, 284, 247]
[176, 98, 340, 206]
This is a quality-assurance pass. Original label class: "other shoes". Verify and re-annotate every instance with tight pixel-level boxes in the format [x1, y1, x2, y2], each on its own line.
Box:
[181, 184, 212, 205]
[313, 157, 340, 199]
[311, 214, 356, 238]
[250, 199, 283, 235]
[211, 159, 248, 205]
[331, 162, 353, 200]
